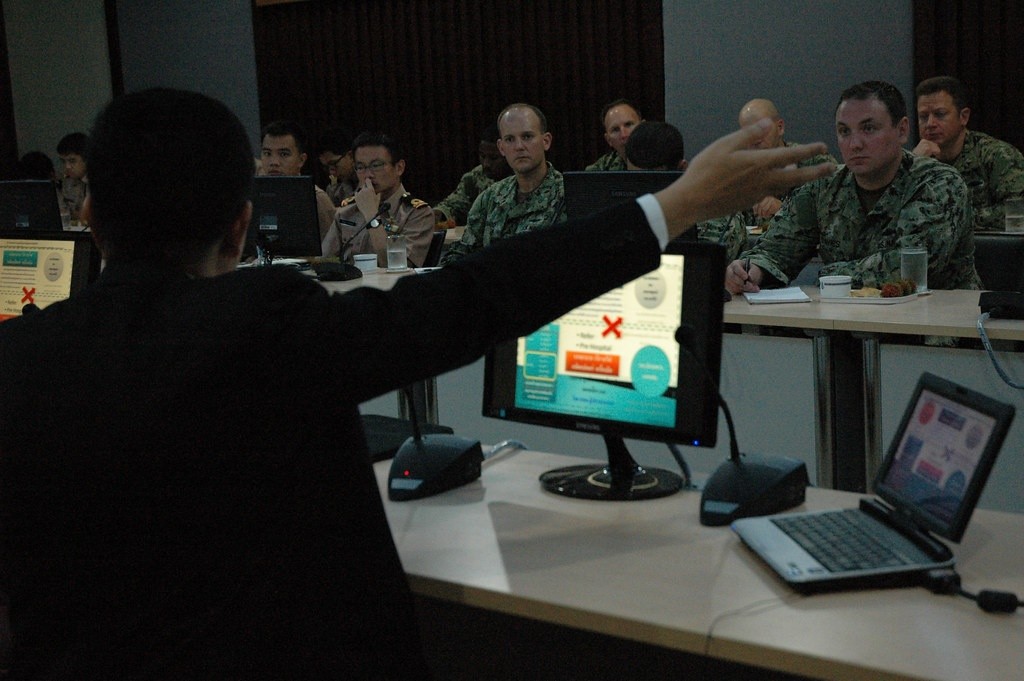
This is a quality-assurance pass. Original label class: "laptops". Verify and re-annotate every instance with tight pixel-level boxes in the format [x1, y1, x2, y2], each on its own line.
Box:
[730, 371, 1015, 597]
[0, 180, 64, 232]
[563, 170, 698, 241]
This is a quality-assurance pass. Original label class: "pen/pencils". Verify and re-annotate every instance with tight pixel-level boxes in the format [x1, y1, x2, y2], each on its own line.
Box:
[356, 258, 376, 261]
[745, 258, 750, 272]
[420, 269, 433, 272]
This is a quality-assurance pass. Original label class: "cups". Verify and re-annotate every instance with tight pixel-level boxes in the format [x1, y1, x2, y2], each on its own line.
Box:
[386, 235, 408, 271]
[900, 246, 928, 293]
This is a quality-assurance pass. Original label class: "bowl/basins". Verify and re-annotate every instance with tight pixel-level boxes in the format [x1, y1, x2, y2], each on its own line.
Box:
[819, 275, 852, 299]
[353, 253, 377, 274]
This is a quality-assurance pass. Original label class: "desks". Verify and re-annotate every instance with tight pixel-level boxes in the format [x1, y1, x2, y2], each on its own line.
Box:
[241, 225, 1024, 681]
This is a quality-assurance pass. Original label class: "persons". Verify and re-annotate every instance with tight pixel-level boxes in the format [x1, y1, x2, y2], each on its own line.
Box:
[912, 76, 1024, 232]
[19, 132, 93, 226]
[725, 80, 984, 298]
[436, 103, 569, 268]
[321, 130, 435, 268]
[0, 87, 837, 681]
[584, 97, 838, 301]
[432, 121, 515, 230]
[260, 120, 337, 242]
[316, 125, 360, 208]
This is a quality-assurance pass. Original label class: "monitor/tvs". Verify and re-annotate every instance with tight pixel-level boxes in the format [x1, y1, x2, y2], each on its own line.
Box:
[481, 239, 728, 501]
[242, 176, 322, 271]
[0, 230, 99, 322]
[972, 232, 1024, 293]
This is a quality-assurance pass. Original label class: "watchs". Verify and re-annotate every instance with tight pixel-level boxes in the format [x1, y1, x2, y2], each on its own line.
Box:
[365, 218, 382, 229]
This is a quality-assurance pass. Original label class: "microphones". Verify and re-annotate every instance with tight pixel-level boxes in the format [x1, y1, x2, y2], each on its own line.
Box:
[314, 202, 391, 282]
[672, 323, 809, 527]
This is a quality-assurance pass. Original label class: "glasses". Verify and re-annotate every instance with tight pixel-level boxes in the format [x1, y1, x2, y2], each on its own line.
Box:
[321, 154, 347, 171]
[352, 160, 397, 173]
